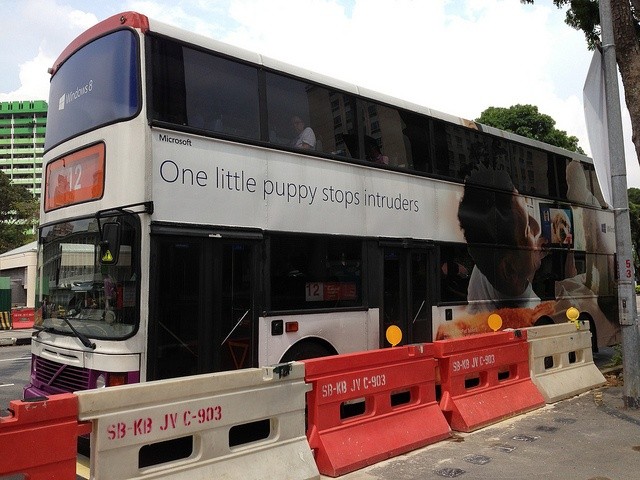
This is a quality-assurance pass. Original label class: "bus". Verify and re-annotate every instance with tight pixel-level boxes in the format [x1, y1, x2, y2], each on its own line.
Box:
[23, 11, 622, 440]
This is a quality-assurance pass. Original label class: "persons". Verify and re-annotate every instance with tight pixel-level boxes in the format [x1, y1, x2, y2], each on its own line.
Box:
[366, 144, 388, 165]
[290, 114, 316, 152]
[458, 163, 544, 309]
